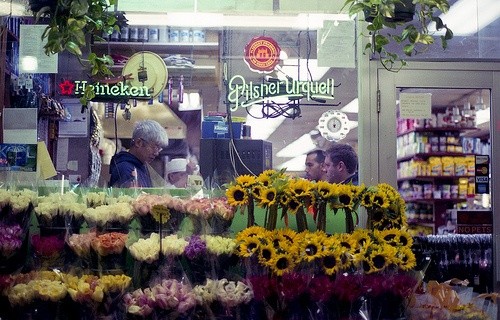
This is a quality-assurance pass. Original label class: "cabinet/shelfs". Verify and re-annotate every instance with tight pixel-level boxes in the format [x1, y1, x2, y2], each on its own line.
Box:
[397, 107, 476, 219]
[96, 24, 220, 77]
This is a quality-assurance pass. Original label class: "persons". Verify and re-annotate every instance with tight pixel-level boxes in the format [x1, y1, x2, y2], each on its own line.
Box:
[108, 120, 196, 189]
[303, 143, 359, 186]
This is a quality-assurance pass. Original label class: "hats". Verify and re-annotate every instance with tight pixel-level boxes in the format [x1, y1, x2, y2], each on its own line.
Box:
[166, 158, 200, 175]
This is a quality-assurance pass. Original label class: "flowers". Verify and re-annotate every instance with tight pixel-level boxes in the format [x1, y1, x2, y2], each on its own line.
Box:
[427, 234, 492, 257]
[0, 171, 416, 320]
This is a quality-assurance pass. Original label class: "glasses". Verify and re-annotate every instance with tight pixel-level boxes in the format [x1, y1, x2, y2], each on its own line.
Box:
[140, 138, 163, 153]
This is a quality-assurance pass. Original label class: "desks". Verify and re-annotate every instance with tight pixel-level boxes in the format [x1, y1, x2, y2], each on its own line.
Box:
[418, 279, 500, 320]
